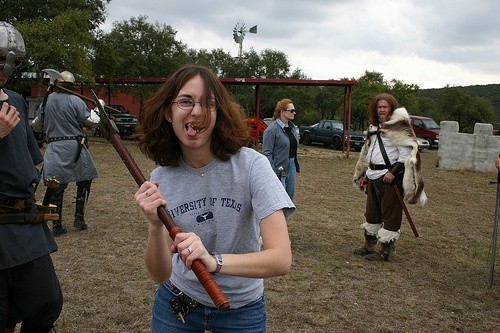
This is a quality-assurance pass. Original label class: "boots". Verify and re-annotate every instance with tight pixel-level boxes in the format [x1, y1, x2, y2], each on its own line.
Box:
[53, 220, 68, 235]
[353, 221, 383, 256]
[74, 213, 87, 229]
[366, 229, 400, 261]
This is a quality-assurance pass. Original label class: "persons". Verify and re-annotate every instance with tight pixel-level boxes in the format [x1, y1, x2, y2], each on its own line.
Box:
[0, 21, 62, 333]
[30, 70, 105, 236]
[354, 93, 428, 262]
[133, 66, 297, 333]
[263, 99, 301, 202]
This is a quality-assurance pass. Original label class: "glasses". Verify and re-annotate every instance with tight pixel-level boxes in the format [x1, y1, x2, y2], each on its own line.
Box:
[173, 98, 219, 111]
[284, 109, 296, 112]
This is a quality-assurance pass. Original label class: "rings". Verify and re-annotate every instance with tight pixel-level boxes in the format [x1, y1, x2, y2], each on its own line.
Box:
[145, 191, 148, 196]
[187, 247, 193, 254]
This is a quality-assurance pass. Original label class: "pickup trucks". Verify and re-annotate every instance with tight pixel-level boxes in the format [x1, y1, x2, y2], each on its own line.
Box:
[299, 119, 366, 153]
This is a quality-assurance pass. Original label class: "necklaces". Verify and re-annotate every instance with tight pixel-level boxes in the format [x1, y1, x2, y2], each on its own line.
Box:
[180, 162, 220, 177]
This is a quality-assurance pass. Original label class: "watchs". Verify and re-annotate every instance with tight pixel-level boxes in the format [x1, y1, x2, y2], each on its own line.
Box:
[212, 252, 223, 275]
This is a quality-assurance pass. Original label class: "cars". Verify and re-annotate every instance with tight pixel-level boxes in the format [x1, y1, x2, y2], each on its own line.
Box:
[263, 117, 274, 126]
[245, 117, 269, 148]
[105, 104, 139, 130]
[415, 137, 429, 152]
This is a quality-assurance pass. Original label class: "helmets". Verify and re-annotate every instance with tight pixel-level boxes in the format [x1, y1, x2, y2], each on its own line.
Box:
[0, 22, 25, 59]
[57, 71, 76, 84]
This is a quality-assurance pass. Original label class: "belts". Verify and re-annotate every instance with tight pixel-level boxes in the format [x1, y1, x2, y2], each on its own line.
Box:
[47, 136, 77, 142]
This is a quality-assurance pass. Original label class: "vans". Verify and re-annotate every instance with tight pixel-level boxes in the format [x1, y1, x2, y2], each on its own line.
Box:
[409, 115, 441, 150]
